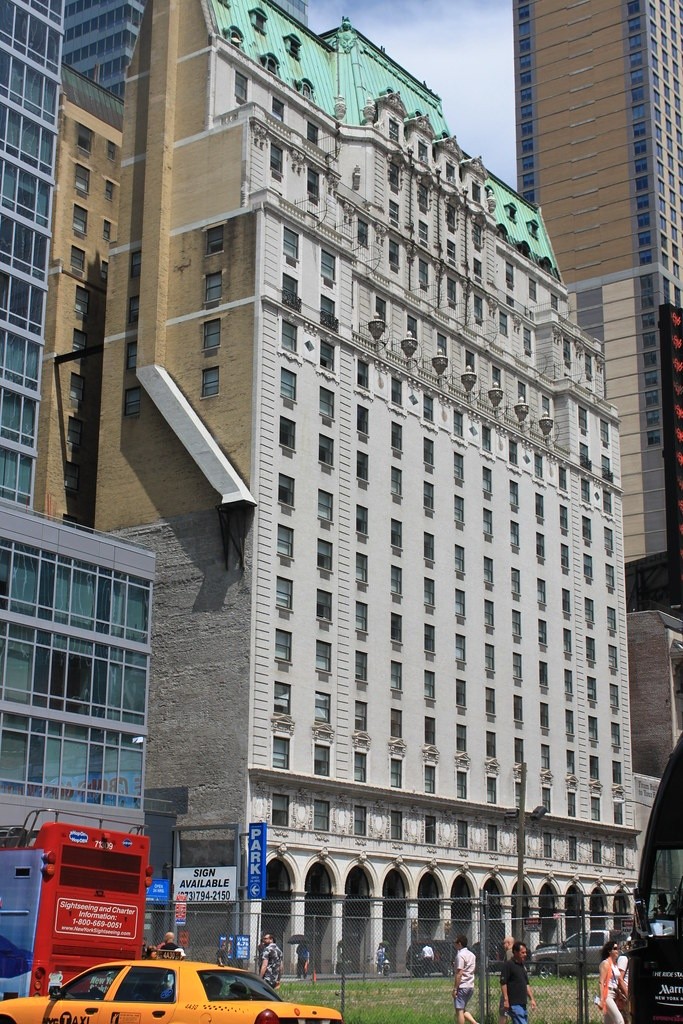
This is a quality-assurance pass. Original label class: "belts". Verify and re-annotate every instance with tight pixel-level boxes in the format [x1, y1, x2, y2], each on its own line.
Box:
[609, 987, 616, 993]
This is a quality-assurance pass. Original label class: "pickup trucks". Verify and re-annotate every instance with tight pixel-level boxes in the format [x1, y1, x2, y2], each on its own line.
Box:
[531, 929, 611, 980]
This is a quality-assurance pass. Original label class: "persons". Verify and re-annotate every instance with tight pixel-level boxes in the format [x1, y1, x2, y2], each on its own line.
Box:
[614, 936, 633, 1024]
[598, 940, 627, 1024]
[216, 942, 228, 966]
[420, 943, 434, 978]
[376, 943, 386, 975]
[498, 937, 515, 1024]
[296, 940, 309, 979]
[451, 935, 481, 1024]
[142, 931, 179, 960]
[500, 942, 537, 1024]
[259, 934, 284, 991]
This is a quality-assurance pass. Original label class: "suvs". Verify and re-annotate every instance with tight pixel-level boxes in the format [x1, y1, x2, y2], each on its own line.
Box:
[405, 940, 461, 978]
[473, 940, 507, 972]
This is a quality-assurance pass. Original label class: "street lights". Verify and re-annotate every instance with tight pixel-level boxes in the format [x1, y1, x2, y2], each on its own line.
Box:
[504, 764, 547, 942]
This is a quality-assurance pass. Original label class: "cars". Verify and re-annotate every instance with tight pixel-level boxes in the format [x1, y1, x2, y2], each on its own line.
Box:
[1, 959, 344, 1024]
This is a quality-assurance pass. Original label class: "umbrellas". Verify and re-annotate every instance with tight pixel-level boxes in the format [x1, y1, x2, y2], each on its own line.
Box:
[287, 935, 311, 944]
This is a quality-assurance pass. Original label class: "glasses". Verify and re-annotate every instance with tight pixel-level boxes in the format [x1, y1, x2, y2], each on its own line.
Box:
[612, 947, 620, 950]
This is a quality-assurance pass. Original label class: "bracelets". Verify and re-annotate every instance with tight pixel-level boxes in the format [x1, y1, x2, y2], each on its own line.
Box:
[453, 990, 457, 991]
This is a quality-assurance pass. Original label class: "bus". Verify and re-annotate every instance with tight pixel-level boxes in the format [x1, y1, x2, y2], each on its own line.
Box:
[0, 806, 154, 1002]
